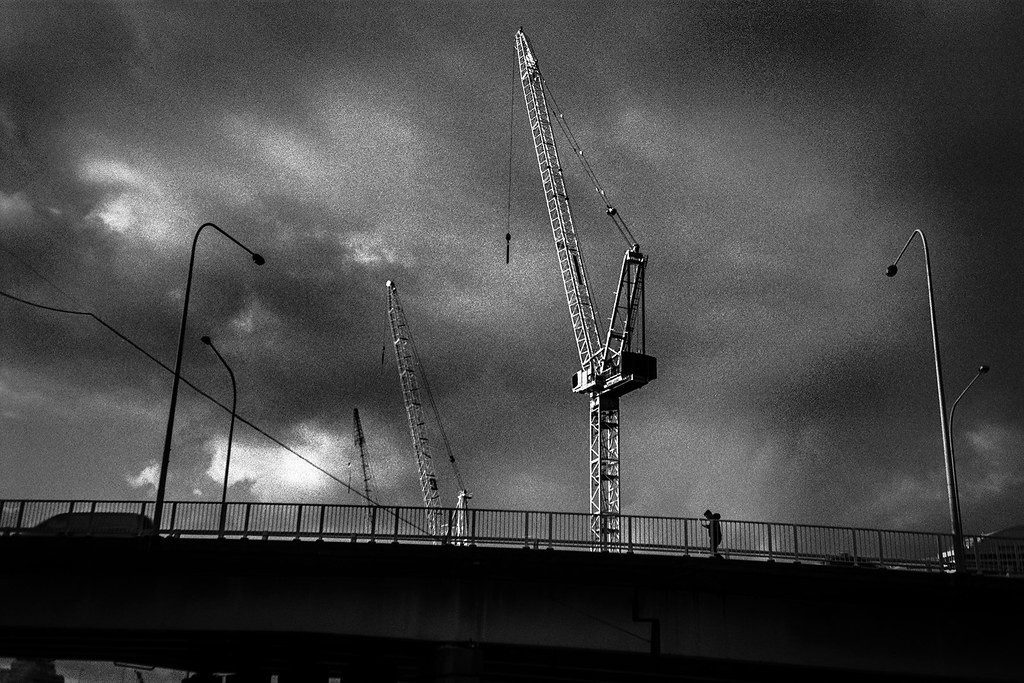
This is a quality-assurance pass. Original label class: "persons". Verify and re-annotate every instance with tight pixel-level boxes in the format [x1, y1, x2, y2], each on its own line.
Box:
[700, 510, 725, 560]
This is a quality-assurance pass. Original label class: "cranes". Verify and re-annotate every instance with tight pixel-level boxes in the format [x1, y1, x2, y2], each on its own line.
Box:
[386, 277, 474, 547]
[513, 24, 659, 555]
[352, 406, 384, 542]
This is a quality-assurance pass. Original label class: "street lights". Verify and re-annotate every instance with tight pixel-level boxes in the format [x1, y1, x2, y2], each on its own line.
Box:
[199, 334, 238, 537]
[885, 228, 966, 576]
[948, 363, 992, 571]
[149, 222, 268, 531]
